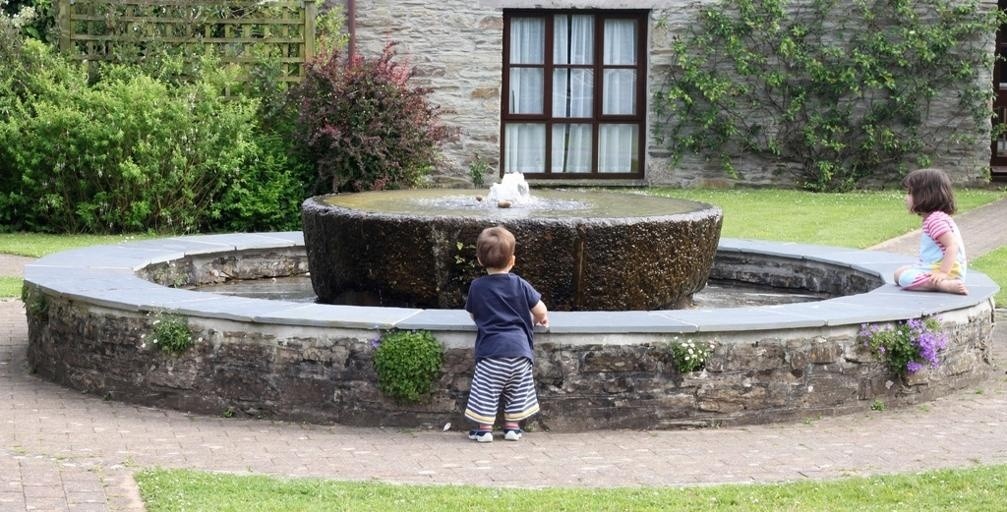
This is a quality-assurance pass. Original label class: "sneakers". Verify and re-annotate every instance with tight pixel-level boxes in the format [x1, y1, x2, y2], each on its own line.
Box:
[504, 428, 522, 441]
[468, 431, 494, 442]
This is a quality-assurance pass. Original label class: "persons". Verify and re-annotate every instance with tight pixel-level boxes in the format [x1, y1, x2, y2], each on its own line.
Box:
[894, 169, 970, 295]
[462, 227, 548, 443]
